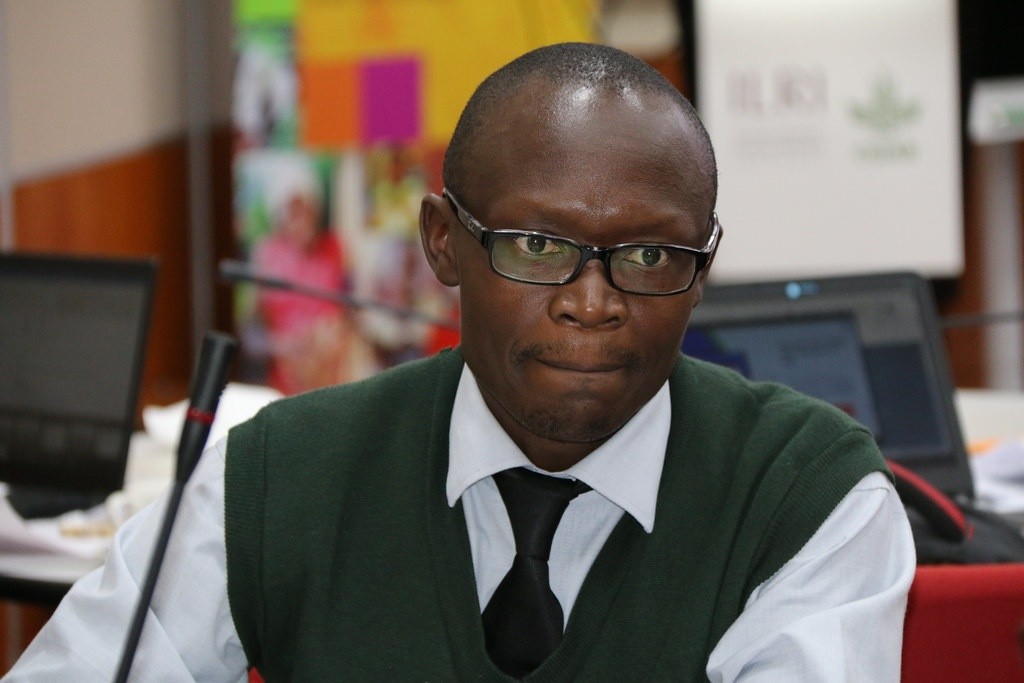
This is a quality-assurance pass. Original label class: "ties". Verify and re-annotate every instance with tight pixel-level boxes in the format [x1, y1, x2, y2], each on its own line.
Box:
[477, 467, 591, 680]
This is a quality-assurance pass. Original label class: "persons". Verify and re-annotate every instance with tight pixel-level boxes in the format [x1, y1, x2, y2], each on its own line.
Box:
[2, 42, 916, 683]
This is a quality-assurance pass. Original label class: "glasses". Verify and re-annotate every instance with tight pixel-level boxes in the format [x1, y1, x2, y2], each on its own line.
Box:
[441, 188, 722, 296]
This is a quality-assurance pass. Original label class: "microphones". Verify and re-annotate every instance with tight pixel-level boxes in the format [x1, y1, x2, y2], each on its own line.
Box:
[112, 252, 458, 682]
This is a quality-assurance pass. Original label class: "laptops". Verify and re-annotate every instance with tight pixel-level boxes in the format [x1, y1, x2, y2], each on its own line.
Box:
[668, 266, 1013, 564]
[0, 248, 160, 608]
